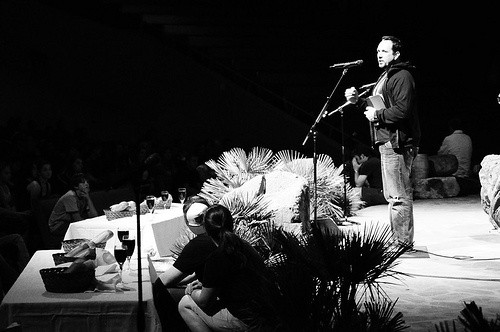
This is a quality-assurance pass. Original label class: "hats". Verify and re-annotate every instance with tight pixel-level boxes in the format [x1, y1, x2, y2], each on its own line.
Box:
[183, 198, 210, 235]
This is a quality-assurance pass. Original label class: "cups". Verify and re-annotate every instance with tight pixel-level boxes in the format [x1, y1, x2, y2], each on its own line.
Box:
[117, 227, 129, 242]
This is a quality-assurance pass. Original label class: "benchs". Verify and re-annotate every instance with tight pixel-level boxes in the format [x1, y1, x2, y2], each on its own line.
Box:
[32, 187, 136, 248]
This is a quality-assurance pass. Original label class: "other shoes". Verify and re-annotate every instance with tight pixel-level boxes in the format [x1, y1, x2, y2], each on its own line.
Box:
[388, 246, 415, 254]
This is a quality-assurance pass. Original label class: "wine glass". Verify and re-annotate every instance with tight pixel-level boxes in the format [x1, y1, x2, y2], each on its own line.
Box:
[179, 188, 186, 205]
[115, 245, 128, 276]
[123, 235, 135, 272]
[162, 191, 169, 211]
[146, 195, 155, 214]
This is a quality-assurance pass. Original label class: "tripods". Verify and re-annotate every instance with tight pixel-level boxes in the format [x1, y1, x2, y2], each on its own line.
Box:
[304, 69, 371, 236]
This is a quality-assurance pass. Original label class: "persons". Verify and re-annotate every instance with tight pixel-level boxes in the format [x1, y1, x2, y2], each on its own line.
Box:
[153, 196, 210, 304]
[1, 113, 472, 286]
[178, 204, 285, 332]
[344, 35, 421, 254]
[48, 173, 97, 238]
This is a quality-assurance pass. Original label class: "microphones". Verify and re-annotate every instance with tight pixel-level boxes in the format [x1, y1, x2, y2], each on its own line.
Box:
[359, 82, 377, 90]
[330, 60, 363, 68]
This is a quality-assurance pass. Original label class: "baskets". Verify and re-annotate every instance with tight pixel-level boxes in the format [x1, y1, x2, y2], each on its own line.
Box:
[103, 208, 132, 220]
[52, 252, 98, 267]
[62, 239, 87, 252]
[41, 269, 95, 293]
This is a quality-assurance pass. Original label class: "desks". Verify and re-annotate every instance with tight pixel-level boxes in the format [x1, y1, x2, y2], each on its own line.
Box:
[60, 202, 192, 257]
[1, 249, 156, 332]
